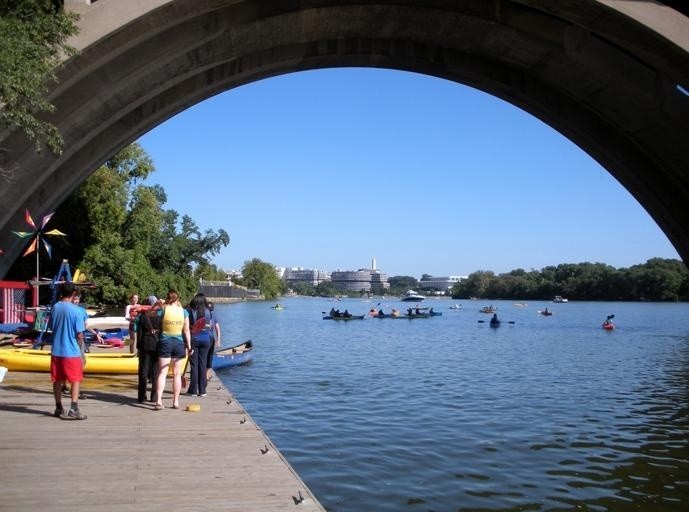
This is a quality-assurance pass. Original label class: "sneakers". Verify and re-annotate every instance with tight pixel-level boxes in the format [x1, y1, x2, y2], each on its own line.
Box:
[55, 409, 87, 420]
[185, 392, 206, 397]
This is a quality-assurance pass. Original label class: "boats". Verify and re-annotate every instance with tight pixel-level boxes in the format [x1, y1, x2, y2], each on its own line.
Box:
[402, 290, 425, 301]
[603, 322, 614, 331]
[0, 323, 130, 344]
[0, 347, 189, 378]
[553, 296, 568, 303]
[210, 339, 253, 370]
[322, 302, 555, 327]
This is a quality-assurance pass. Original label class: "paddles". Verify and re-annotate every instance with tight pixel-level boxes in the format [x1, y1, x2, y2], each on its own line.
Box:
[601, 315, 614, 327]
[478, 321, 515, 323]
[181, 317, 205, 387]
[374, 302, 381, 310]
[321, 311, 330, 314]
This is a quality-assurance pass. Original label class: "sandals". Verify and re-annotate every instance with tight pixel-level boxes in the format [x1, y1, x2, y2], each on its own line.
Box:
[155, 404, 179, 409]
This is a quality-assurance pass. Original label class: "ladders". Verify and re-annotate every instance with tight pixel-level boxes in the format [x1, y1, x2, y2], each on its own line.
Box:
[33, 259, 89, 352]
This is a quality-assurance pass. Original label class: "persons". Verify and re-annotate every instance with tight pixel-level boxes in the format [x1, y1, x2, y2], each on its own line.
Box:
[430, 308, 434, 313]
[343, 310, 349, 317]
[377, 303, 384, 315]
[416, 309, 420, 314]
[334, 309, 340, 317]
[544, 308, 549, 315]
[61, 289, 104, 400]
[124, 289, 220, 410]
[491, 314, 497, 321]
[48, 280, 88, 420]
[330, 308, 335, 315]
[606, 315, 613, 325]
